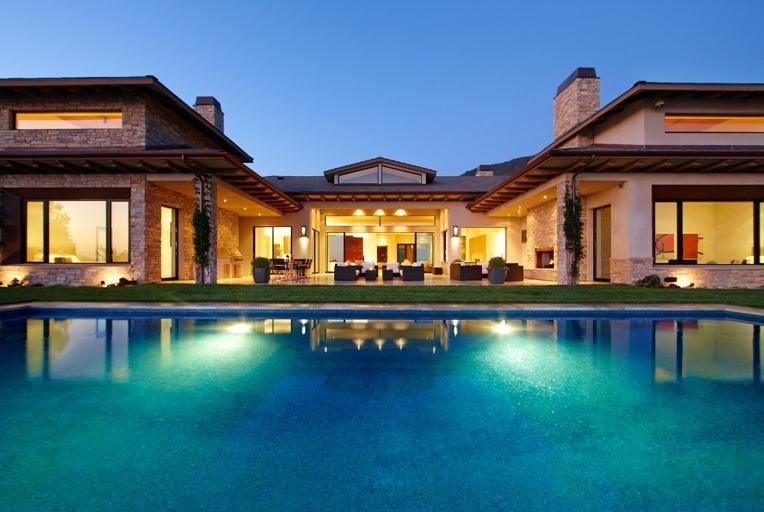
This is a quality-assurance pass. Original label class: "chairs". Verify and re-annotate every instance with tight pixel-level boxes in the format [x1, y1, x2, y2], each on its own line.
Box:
[270, 258, 312, 280]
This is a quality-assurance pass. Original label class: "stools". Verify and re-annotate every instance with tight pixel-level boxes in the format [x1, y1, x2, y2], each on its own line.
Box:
[432, 266, 442, 275]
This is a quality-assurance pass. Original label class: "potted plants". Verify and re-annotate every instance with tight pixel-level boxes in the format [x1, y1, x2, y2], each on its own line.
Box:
[486, 257, 504, 284]
[251, 257, 270, 283]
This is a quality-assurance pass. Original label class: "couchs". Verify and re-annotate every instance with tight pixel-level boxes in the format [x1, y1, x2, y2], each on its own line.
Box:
[334, 258, 424, 281]
[451, 262, 523, 281]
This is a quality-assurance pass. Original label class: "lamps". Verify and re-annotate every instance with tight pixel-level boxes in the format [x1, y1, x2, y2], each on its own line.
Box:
[301, 225, 306, 236]
[453, 225, 458, 236]
